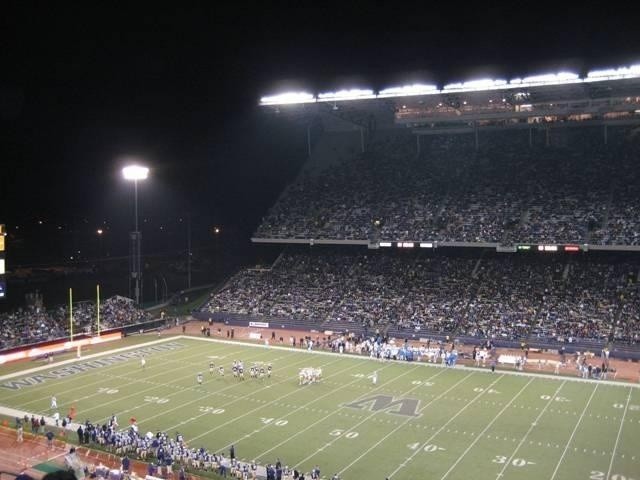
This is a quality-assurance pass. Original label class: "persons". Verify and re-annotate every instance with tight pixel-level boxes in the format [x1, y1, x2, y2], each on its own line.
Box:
[13, 393, 340, 479]
[198, 119, 639, 385]
[0, 287, 192, 369]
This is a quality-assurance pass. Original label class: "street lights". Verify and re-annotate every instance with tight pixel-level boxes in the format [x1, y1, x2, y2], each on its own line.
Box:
[123, 166, 147, 303]
